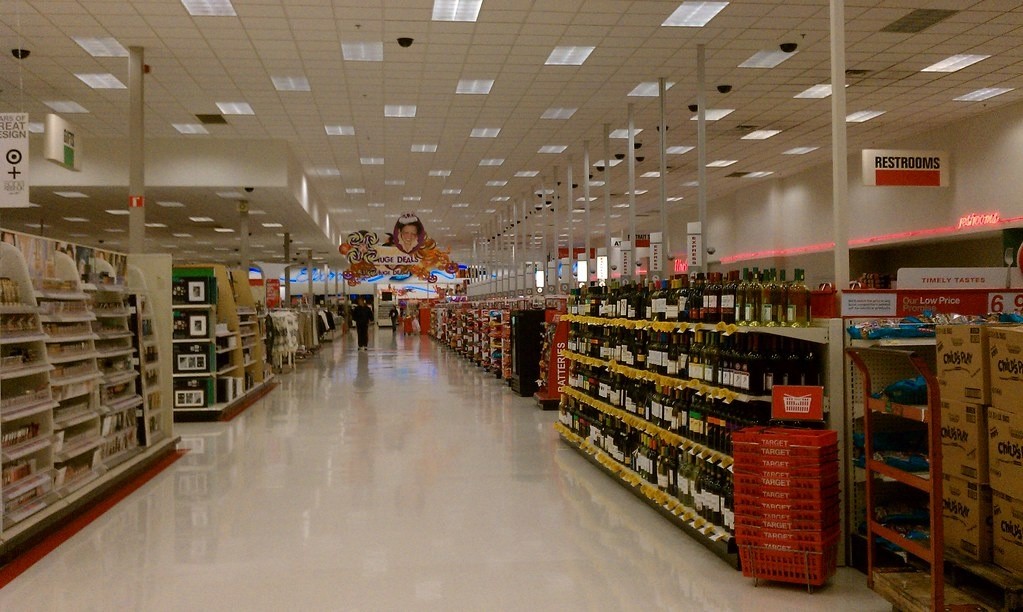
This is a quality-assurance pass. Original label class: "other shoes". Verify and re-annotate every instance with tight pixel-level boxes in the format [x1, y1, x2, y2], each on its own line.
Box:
[358, 347, 368, 351]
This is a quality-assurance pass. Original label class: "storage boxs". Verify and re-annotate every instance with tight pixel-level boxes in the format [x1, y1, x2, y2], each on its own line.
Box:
[731, 424, 840, 588]
[935, 323, 1023, 582]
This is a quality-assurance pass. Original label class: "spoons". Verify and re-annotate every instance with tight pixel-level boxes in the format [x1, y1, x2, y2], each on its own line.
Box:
[1005, 248, 1013, 288]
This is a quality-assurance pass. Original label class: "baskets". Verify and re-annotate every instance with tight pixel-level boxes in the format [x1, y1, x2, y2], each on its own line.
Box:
[731, 427, 840, 585]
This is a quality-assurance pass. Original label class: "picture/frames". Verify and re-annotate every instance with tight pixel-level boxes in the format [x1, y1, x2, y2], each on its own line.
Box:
[172, 277, 210, 408]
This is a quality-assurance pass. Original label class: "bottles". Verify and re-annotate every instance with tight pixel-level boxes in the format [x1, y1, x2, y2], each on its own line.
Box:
[559, 266, 820, 532]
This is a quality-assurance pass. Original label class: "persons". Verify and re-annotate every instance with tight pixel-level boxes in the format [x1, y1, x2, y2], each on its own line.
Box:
[402, 314, 421, 335]
[389, 306, 398, 334]
[353, 298, 374, 351]
[398, 220, 419, 253]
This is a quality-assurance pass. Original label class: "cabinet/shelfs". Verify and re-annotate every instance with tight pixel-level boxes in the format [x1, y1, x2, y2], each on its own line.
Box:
[216, 264, 275, 405]
[1, 241, 171, 531]
[533, 297, 570, 411]
[556, 317, 843, 573]
[428, 297, 541, 382]
[845, 337, 1023, 583]
[172, 304, 221, 412]
